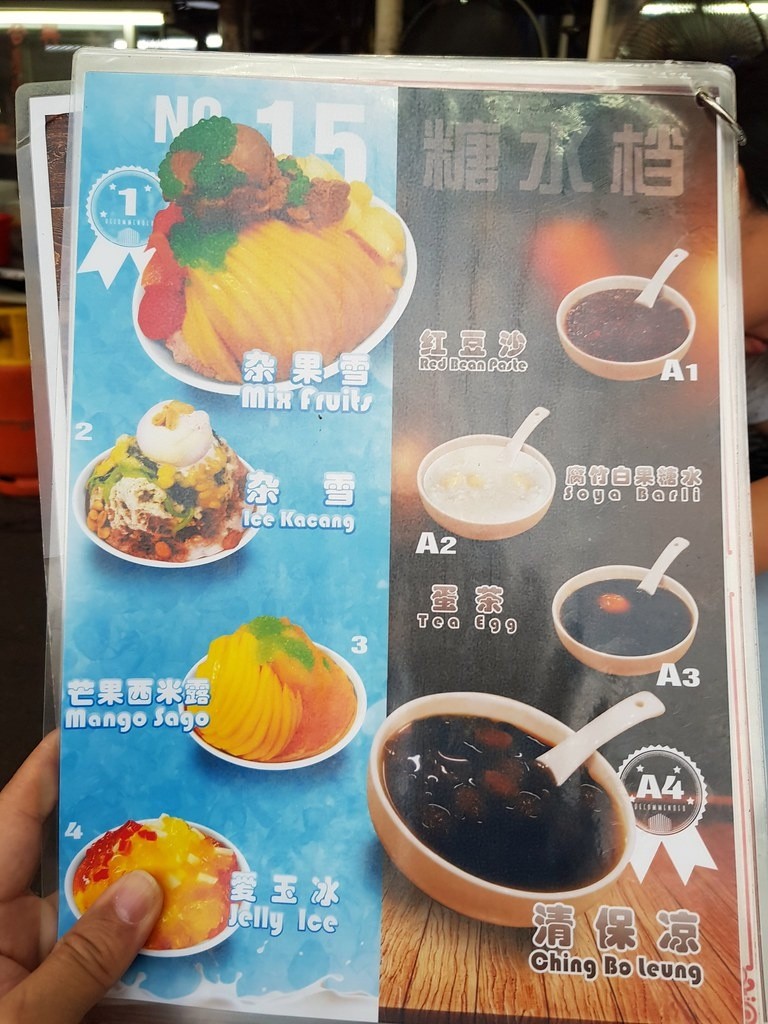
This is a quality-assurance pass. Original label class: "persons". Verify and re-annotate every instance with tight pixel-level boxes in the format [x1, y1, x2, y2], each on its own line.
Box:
[583, 11, 768, 805]
[0, 727, 164, 1024]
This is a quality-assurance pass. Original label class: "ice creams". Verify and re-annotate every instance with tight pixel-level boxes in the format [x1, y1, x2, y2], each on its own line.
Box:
[158, 116, 350, 229]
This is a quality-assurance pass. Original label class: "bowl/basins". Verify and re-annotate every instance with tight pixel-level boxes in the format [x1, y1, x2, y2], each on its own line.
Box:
[556, 275, 696, 382]
[416, 434, 556, 541]
[551, 565, 699, 676]
[366, 692, 636, 929]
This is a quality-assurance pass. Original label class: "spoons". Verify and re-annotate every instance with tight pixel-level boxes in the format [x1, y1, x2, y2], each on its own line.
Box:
[535, 690, 666, 787]
[636, 537, 690, 595]
[634, 248, 689, 310]
[498, 406, 550, 467]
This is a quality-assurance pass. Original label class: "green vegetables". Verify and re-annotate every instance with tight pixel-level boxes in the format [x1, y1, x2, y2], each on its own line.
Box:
[87, 456, 194, 536]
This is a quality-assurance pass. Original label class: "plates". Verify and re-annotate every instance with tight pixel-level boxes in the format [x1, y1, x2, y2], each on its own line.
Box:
[73, 446, 269, 569]
[178, 641, 368, 770]
[64, 819, 252, 958]
[132, 195, 418, 397]
